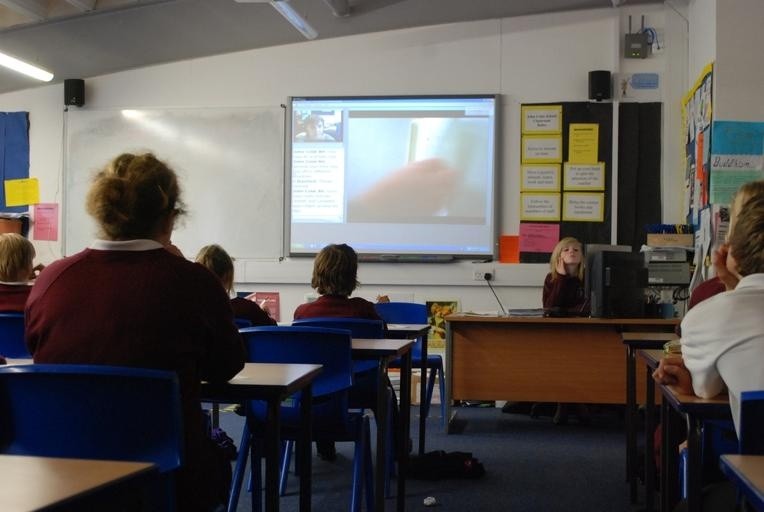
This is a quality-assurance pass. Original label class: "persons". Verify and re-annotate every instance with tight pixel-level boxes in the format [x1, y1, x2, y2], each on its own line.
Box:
[345, 155, 463, 221]
[1, 232, 38, 283]
[428, 306, 451, 336]
[542, 234, 590, 434]
[22, 150, 247, 507]
[685, 179, 764, 307]
[294, 115, 337, 144]
[293, 242, 415, 461]
[188, 243, 278, 326]
[430, 304, 443, 337]
[653, 195, 763, 447]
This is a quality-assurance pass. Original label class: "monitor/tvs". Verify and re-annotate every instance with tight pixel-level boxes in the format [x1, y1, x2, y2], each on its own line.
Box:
[585, 244, 633, 300]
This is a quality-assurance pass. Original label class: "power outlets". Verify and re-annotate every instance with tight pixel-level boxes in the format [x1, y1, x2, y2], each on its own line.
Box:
[474, 269, 495, 281]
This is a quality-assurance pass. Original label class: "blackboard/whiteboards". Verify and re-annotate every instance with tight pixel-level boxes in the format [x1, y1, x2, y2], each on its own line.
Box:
[61, 104, 287, 261]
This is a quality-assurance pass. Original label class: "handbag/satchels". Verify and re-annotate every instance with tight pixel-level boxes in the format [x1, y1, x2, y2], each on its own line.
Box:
[398, 451, 487, 480]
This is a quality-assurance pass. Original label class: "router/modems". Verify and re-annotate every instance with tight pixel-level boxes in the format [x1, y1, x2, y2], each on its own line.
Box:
[625, 15, 648, 59]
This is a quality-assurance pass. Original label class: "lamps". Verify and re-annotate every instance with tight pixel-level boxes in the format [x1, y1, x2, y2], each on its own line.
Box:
[1, 52, 54, 83]
[269, 0, 320, 42]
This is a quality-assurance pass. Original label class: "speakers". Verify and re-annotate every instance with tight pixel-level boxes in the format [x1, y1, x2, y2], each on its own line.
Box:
[64, 79, 85, 105]
[588, 70, 611, 99]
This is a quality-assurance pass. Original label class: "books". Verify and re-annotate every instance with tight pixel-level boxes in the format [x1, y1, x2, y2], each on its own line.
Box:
[509, 308, 545, 318]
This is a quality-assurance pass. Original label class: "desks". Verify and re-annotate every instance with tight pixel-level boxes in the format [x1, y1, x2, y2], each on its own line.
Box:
[620, 332, 764, 512]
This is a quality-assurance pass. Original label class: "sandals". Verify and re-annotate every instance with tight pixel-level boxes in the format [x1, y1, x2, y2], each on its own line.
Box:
[553, 406, 564, 424]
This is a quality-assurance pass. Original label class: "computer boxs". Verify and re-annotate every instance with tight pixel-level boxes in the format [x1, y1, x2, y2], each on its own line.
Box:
[590, 250, 645, 318]
